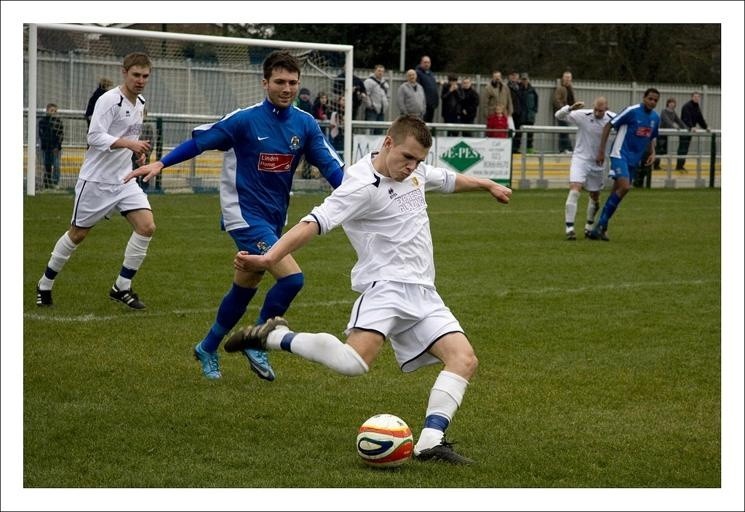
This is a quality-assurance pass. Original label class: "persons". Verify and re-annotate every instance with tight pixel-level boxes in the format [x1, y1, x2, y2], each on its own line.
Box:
[553, 96, 619, 242]
[33, 52, 155, 311]
[549, 70, 579, 154]
[672, 92, 711, 171]
[223, 114, 514, 467]
[82, 76, 114, 149]
[651, 98, 691, 171]
[291, 55, 540, 180]
[38, 104, 64, 189]
[123, 49, 346, 381]
[588, 88, 661, 241]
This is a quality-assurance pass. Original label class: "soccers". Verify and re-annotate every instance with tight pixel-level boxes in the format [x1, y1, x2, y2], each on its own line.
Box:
[357, 414, 413, 467]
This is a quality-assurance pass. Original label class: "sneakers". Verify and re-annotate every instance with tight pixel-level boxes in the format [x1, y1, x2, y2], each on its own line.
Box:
[224, 316, 289, 351]
[36, 282, 53, 308]
[567, 229, 610, 241]
[413, 443, 475, 465]
[193, 343, 222, 380]
[242, 349, 275, 381]
[108, 283, 146, 310]
